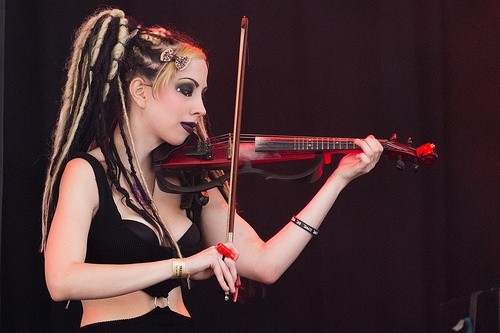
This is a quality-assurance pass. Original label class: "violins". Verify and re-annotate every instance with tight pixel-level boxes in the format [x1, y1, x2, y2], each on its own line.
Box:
[149, 131, 437, 194]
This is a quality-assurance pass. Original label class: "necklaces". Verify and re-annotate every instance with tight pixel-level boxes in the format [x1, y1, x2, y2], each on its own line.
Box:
[125, 170, 158, 209]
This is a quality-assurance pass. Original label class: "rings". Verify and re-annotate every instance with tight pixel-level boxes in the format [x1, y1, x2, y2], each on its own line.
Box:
[217, 243, 234, 261]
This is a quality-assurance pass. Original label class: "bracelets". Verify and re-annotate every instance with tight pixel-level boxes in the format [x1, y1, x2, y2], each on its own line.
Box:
[291, 216, 319, 236]
[172, 257, 186, 278]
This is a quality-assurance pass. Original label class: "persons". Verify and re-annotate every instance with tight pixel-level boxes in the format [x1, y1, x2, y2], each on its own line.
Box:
[40, 8, 384, 333]
